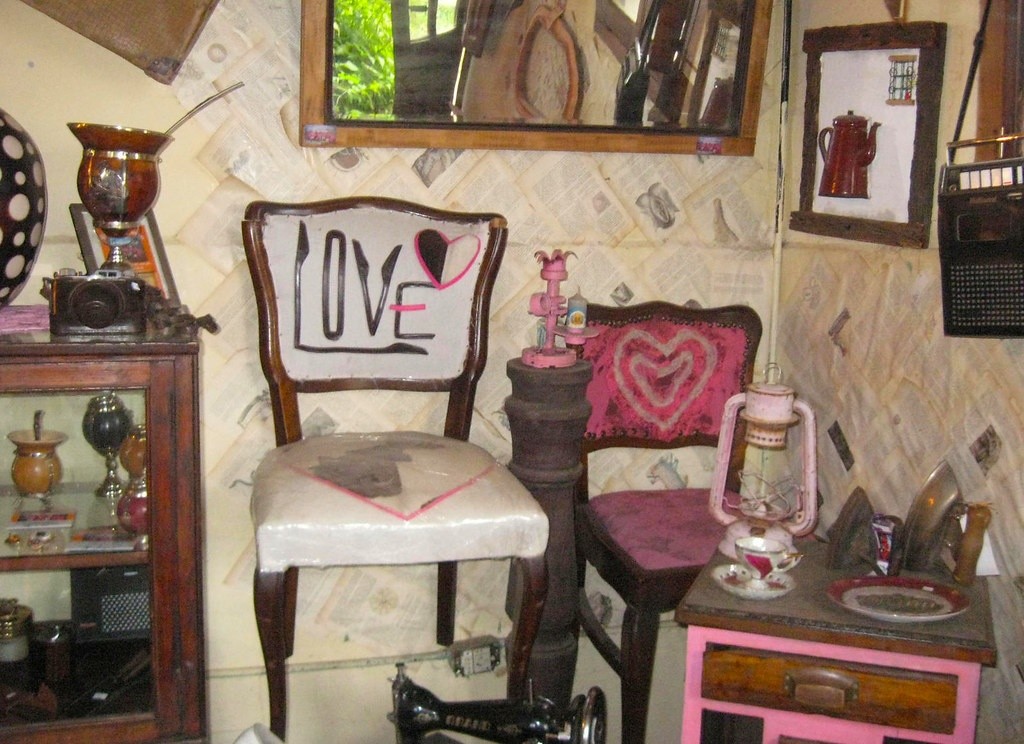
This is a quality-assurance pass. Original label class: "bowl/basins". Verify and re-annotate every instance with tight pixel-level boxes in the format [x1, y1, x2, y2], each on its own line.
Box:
[726, 519, 801, 547]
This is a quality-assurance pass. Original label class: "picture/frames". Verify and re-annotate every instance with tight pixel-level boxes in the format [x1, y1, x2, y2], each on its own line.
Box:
[789, 14, 944, 249]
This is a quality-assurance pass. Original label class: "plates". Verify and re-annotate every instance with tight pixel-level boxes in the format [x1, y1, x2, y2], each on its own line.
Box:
[718, 539, 801, 571]
[711, 564, 795, 600]
[826, 576, 971, 623]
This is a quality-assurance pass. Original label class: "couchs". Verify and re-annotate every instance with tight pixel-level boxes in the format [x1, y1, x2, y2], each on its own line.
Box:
[244, 194, 546, 741]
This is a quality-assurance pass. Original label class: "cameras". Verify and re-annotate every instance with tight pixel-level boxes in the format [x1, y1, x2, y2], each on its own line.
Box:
[45, 268, 148, 344]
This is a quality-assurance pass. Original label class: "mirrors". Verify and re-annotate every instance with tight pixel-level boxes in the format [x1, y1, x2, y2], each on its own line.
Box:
[302, 2, 773, 155]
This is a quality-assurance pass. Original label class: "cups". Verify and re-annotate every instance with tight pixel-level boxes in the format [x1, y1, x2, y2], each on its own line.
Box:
[33, 621, 78, 684]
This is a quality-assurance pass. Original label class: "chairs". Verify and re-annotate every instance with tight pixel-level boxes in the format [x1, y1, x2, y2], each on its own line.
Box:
[572, 301, 820, 744]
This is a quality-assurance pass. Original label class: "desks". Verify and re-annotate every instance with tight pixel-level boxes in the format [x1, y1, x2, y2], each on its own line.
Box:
[672, 539, 1000, 744]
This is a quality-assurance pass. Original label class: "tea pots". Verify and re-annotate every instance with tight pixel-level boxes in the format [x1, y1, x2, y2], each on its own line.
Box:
[817, 110, 881, 199]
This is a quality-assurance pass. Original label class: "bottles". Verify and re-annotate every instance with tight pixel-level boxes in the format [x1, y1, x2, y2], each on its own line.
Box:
[566, 287, 587, 329]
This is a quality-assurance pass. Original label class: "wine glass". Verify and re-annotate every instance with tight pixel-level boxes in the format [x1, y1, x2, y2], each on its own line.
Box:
[736, 536, 789, 589]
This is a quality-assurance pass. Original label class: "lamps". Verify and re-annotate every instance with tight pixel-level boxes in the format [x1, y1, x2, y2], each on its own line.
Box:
[705, 363, 821, 602]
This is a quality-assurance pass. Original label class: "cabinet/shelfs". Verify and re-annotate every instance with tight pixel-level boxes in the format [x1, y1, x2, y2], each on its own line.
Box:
[1, 304, 211, 742]
